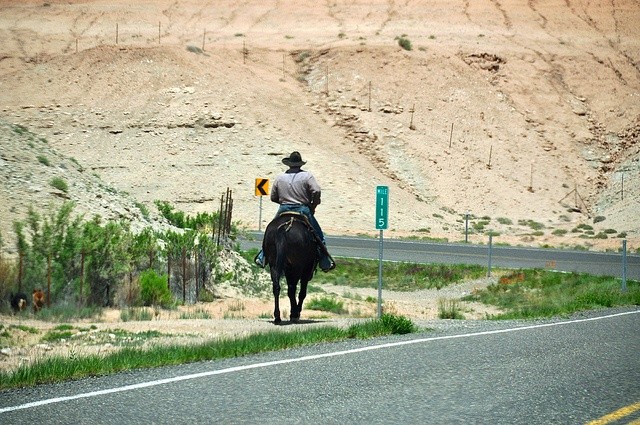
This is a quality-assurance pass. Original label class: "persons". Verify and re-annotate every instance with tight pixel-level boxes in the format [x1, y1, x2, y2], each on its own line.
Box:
[255, 151, 336, 273]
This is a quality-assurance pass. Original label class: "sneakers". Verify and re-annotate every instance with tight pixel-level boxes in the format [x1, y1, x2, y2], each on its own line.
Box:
[323, 263, 334, 272]
[256, 258, 263, 263]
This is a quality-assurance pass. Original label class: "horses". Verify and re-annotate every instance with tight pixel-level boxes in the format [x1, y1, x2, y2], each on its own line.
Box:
[263, 214, 319, 325]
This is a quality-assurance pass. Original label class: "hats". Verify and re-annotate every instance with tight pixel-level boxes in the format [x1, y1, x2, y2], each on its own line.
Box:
[282, 151, 307, 167]
